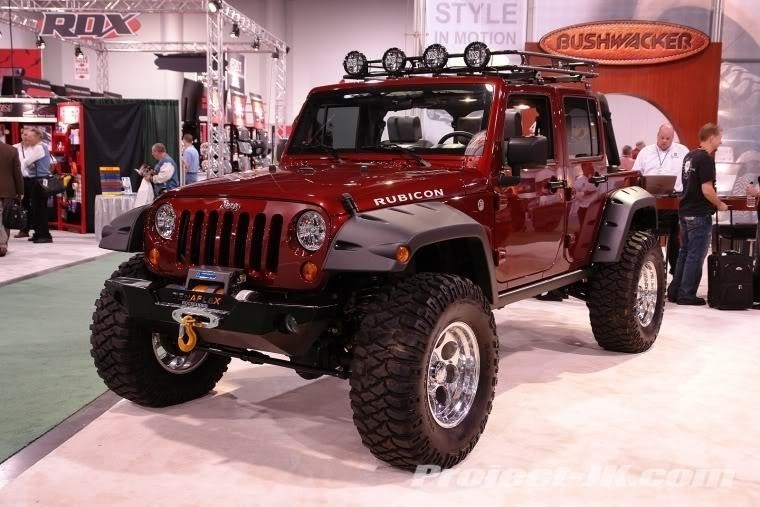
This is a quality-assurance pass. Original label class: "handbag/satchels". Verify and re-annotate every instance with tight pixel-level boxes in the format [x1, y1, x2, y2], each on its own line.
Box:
[35, 174, 66, 197]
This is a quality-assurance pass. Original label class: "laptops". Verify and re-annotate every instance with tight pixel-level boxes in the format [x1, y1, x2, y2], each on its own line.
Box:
[638, 176, 678, 197]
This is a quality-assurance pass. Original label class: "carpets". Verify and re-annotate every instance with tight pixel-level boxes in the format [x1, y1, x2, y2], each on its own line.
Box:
[0, 249, 144, 463]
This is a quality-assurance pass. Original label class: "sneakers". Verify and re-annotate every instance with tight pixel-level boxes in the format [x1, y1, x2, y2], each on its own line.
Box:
[0, 230, 53, 257]
[667, 291, 705, 306]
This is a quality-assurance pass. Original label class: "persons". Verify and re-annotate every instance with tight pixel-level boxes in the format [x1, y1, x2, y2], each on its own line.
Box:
[745, 175, 760, 310]
[619, 146, 636, 170]
[144, 142, 180, 195]
[632, 140, 647, 158]
[0, 130, 25, 256]
[182, 133, 200, 186]
[23, 128, 53, 242]
[632, 123, 691, 273]
[11, 126, 58, 237]
[666, 122, 729, 306]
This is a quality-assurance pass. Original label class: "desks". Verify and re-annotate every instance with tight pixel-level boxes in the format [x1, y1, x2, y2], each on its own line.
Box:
[95, 193, 137, 243]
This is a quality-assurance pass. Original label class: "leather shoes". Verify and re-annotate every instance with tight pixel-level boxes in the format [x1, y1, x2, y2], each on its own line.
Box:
[539, 291, 568, 301]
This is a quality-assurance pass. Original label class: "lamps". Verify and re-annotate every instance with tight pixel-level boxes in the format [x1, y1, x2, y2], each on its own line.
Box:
[75, 45, 84, 60]
[208, 0, 282, 60]
[34, 33, 46, 49]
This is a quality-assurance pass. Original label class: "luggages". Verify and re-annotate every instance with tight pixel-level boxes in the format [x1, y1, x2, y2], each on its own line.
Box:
[707, 205, 753, 310]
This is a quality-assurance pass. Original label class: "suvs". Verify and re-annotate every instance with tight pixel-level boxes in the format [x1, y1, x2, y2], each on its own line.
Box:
[89, 37, 685, 477]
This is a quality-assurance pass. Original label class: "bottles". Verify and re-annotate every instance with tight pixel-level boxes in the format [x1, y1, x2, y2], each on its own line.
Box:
[747, 181, 755, 207]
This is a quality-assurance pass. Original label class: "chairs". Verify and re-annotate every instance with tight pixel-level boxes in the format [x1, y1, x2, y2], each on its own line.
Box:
[713, 194, 760, 252]
[379, 108, 523, 147]
